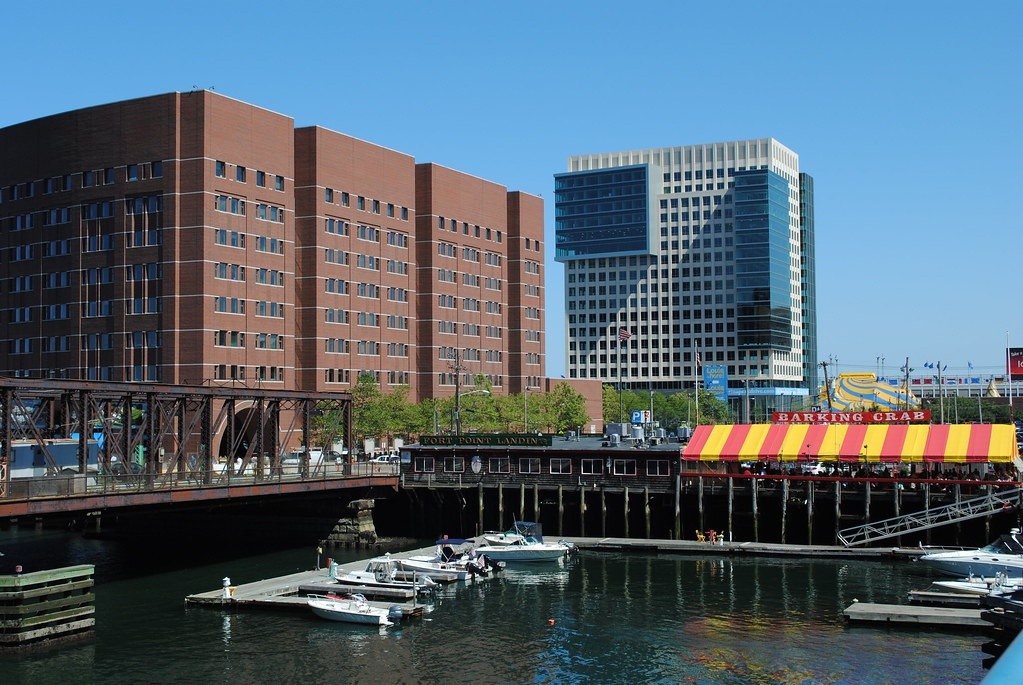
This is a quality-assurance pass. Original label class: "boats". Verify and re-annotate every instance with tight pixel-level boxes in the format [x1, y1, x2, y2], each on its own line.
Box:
[475, 542, 575, 561]
[482, 533, 524, 545]
[334, 551, 444, 595]
[409, 556, 494, 578]
[933, 581, 1018, 594]
[306, 594, 394, 627]
[395, 559, 479, 580]
[919, 528, 1023, 578]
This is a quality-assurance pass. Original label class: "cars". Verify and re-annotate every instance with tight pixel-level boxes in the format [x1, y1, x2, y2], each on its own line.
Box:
[323, 451, 343, 460]
[368, 454, 399, 464]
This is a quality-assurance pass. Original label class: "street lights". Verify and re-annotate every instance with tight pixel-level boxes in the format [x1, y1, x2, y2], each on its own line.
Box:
[976, 392, 982, 424]
[524, 385, 531, 433]
[650, 391, 654, 436]
[458, 389, 490, 435]
[688, 392, 691, 428]
[751, 397, 757, 424]
[780, 391, 784, 424]
[952, 389, 958, 424]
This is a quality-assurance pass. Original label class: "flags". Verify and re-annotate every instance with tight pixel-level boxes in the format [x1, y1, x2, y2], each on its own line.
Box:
[618, 329, 633, 342]
